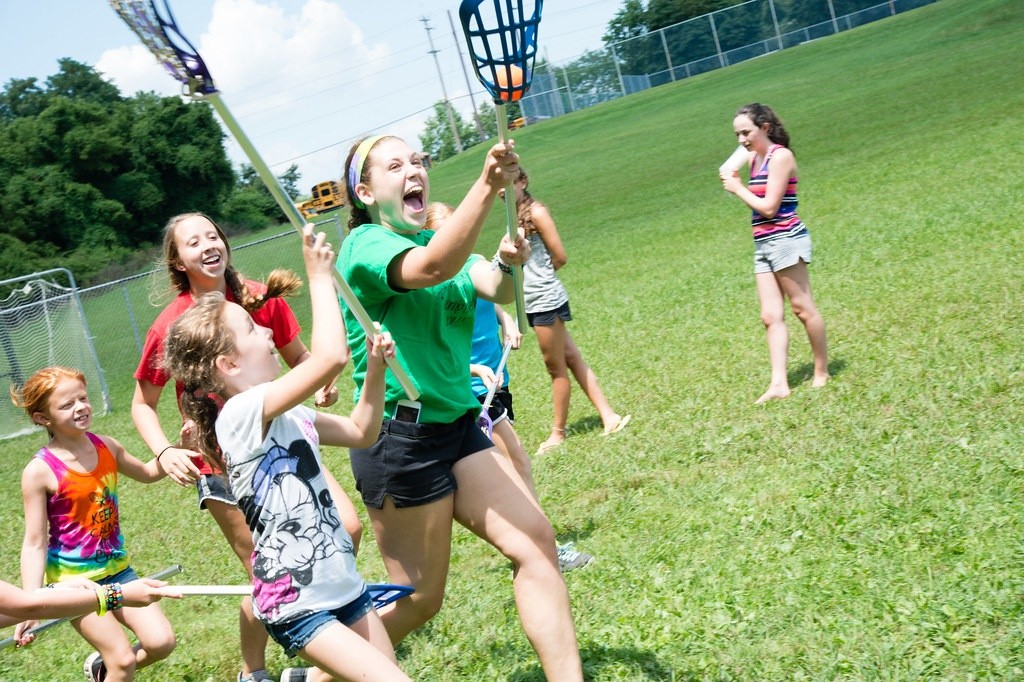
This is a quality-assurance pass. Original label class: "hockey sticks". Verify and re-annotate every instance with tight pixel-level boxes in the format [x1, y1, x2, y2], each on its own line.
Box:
[458, 0, 546, 335]
[111, 0, 420, 400]
[159, 584, 414, 609]
[0, 564, 183, 649]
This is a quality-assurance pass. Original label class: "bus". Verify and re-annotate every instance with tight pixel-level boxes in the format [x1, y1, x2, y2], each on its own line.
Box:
[311, 180, 343, 214]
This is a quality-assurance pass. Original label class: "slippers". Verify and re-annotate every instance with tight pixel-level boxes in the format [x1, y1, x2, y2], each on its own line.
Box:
[535, 438, 570, 456]
[601, 414, 633, 439]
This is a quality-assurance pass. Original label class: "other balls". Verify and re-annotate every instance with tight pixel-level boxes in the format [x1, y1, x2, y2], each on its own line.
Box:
[496, 65, 522, 101]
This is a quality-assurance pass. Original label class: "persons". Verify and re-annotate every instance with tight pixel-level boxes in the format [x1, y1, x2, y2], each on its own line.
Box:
[420, 203, 598, 574]
[719, 102, 831, 405]
[491, 166, 630, 458]
[1, 576, 183, 630]
[322, 132, 586, 682]
[10, 366, 197, 679]
[166, 224, 418, 682]
[129, 213, 367, 682]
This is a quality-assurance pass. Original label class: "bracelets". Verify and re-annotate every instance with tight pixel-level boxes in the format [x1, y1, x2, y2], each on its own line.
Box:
[492, 248, 527, 276]
[93, 579, 123, 619]
[156, 444, 174, 463]
[47, 582, 54, 590]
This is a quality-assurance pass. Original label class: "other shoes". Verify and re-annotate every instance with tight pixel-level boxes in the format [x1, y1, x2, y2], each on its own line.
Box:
[279, 667, 307, 682]
[558, 543, 593, 574]
[237, 669, 275, 682]
[82, 651, 107, 682]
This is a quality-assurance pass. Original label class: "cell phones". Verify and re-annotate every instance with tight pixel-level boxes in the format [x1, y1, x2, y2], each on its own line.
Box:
[392, 398, 422, 424]
